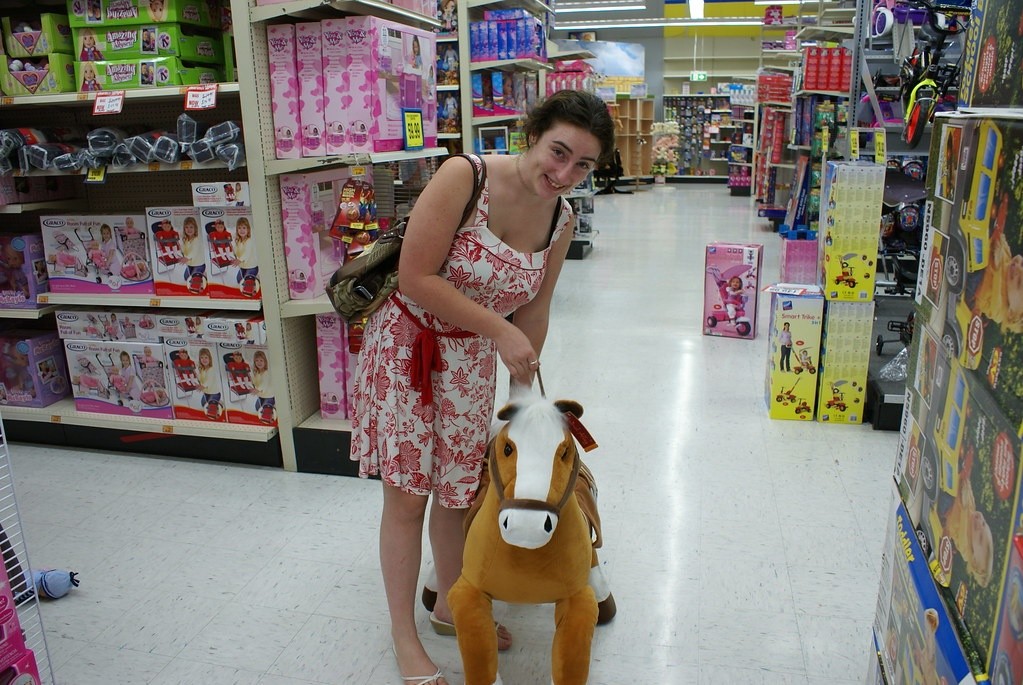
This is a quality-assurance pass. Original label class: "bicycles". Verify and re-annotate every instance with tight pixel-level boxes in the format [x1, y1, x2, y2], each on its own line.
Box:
[879, 0, 970, 149]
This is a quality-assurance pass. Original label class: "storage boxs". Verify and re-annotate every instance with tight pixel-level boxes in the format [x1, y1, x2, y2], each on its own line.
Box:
[1, 0, 1023, 685]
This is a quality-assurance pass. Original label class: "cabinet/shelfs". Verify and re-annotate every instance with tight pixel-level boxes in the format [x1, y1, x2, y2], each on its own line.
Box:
[602, 94, 655, 184]
[845, 0, 971, 431]
[536, 38, 599, 261]
[751, 24, 800, 228]
[0, 0, 457, 481]
[726, 74, 756, 196]
[779, 0, 857, 239]
[394, 0, 556, 186]
[661, 95, 734, 182]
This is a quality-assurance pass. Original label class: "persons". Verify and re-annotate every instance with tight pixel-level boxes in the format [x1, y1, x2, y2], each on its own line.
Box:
[6, 245, 29, 292]
[802, 350, 808, 365]
[429, 66, 435, 86]
[349, 90, 615, 685]
[142, 346, 158, 364]
[100, 224, 120, 277]
[726, 276, 746, 325]
[186, 317, 205, 339]
[444, 92, 457, 117]
[156, 216, 206, 281]
[118, 350, 141, 402]
[13, 349, 34, 392]
[148, 0, 168, 22]
[237, 322, 255, 345]
[946, 475, 994, 588]
[443, 43, 459, 66]
[77, 27, 105, 92]
[111, 314, 130, 334]
[175, 349, 221, 408]
[209, 216, 259, 284]
[779, 322, 792, 373]
[412, 35, 422, 69]
[126, 217, 141, 234]
[229, 349, 275, 411]
[226, 183, 245, 207]
[978, 231, 1023, 333]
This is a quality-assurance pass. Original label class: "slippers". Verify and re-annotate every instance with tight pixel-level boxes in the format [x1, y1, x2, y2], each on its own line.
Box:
[392, 643, 445, 685]
[429, 611, 513, 650]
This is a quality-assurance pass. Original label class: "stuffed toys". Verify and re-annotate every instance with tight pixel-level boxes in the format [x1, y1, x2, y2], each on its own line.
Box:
[418, 399, 600, 685]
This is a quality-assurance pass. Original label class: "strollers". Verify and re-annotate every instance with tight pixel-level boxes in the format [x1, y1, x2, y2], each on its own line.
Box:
[74, 227, 113, 284]
[835, 253, 857, 288]
[98, 314, 119, 341]
[96, 353, 133, 406]
[794, 398, 811, 414]
[790, 345, 816, 374]
[775, 377, 800, 403]
[706, 264, 752, 336]
[826, 379, 849, 412]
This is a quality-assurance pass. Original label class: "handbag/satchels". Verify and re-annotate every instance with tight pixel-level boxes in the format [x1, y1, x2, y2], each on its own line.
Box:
[325, 152, 487, 326]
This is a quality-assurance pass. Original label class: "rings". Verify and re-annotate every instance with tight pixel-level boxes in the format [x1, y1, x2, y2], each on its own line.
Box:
[530, 360, 537, 365]
